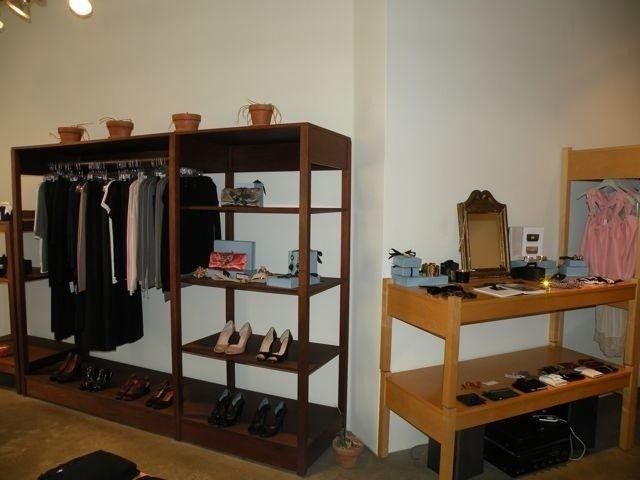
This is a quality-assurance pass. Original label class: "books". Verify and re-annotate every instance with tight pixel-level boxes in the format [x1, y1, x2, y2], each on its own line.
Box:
[473, 283, 546, 298]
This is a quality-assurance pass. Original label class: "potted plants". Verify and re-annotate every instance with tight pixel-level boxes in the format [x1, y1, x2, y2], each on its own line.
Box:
[240, 99, 284, 124]
[331, 406, 365, 471]
[166, 112, 202, 131]
[98, 114, 136, 139]
[48, 121, 92, 142]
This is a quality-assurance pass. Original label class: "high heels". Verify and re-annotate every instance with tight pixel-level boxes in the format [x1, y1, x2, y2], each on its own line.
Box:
[223, 322, 253, 355]
[49, 352, 73, 382]
[259, 400, 286, 438]
[113, 373, 139, 400]
[255, 326, 277, 361]
[123, 371, 151, 402]
[89, 367, 113, 393]
[221, 391, 245, 427]
[78, 365, 100, 391]
[57, 353, 78, 384]
[153, 384, 176, 411]
[266, 328, 294, 362]
[207, 388, 231, 426]
[144, 381, 168, 408]
[213, 319, 235, 354]
[247, 396, 271, 436]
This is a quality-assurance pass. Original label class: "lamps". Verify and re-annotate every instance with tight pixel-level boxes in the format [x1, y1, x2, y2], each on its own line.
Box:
[6, 1, 50, 24]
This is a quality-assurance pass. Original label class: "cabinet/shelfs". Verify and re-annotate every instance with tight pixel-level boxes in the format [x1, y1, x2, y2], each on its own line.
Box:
[550, 144, 640, 451]
[10, 122, 352, 478]
[0, 209, 65, 382]
[378, 244, 640, 480]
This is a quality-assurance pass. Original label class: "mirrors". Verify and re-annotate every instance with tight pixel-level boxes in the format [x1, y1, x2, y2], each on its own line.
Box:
[456, 189, 512, 285]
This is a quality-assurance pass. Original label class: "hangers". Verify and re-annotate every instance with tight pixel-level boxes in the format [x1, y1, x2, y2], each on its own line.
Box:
[575, 179, 636, 208]
[614, 179, 640, 203]
[47, 153, 170, 193]
